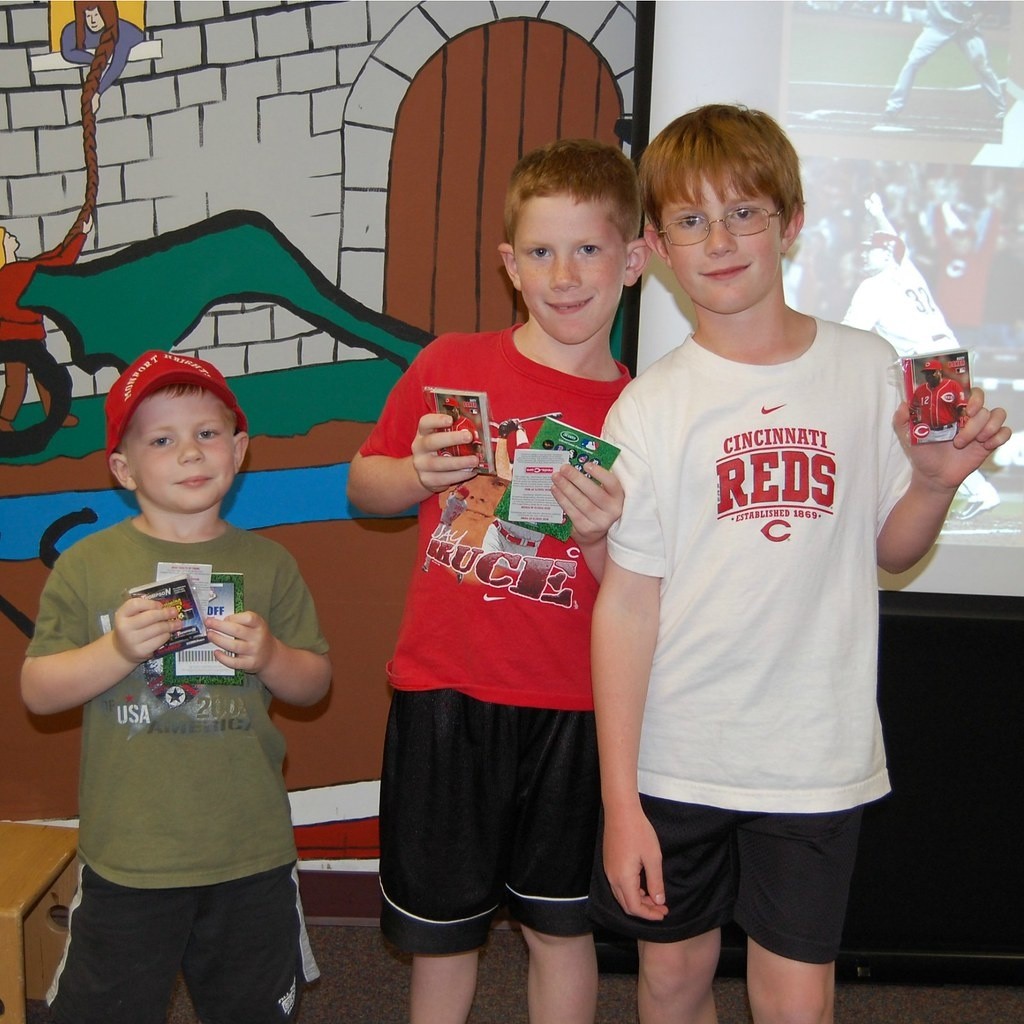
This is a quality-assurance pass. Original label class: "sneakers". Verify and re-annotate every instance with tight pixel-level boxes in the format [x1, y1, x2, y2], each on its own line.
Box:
[955, 484, 1000, 520]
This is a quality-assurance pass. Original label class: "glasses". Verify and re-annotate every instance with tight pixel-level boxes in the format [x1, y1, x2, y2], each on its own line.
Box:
[658, 205, 787, 246]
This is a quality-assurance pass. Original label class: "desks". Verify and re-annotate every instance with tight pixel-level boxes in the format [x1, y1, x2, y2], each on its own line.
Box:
[0, 820, 82, 1024]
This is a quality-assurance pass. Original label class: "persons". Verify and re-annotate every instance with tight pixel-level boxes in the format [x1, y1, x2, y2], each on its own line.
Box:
[839, 1, 1023, 519]
[347, 139, 648, 1024]
[421, 421, 547, 583]
[907, 360, 968, 441]
[20, 354, 331, 1024]
[592, 104, 1012, 1024]
[440, 398, 484, 467]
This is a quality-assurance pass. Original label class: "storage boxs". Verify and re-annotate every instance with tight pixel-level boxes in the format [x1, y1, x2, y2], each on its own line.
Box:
[129, 573, 210, 660]
[431, 387, 496, 476]
[902, 348, 971, 445]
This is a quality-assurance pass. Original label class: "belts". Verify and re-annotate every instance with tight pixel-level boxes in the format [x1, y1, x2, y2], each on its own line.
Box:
[930, 424, 952, 431]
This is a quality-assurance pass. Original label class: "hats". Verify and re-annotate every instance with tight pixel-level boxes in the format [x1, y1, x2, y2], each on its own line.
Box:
[859, 232, 905, 261]
[921, 360, 942, 372]
[443, 398, 459, 407]
[105, 350, 247, 461]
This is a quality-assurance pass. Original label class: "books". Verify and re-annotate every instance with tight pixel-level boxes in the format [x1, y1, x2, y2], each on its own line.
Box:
[430, 388, 494, 474]
[902, 349, 972, 444]
[494, 418, 622, 542]
[129, 573, 245, 685]
